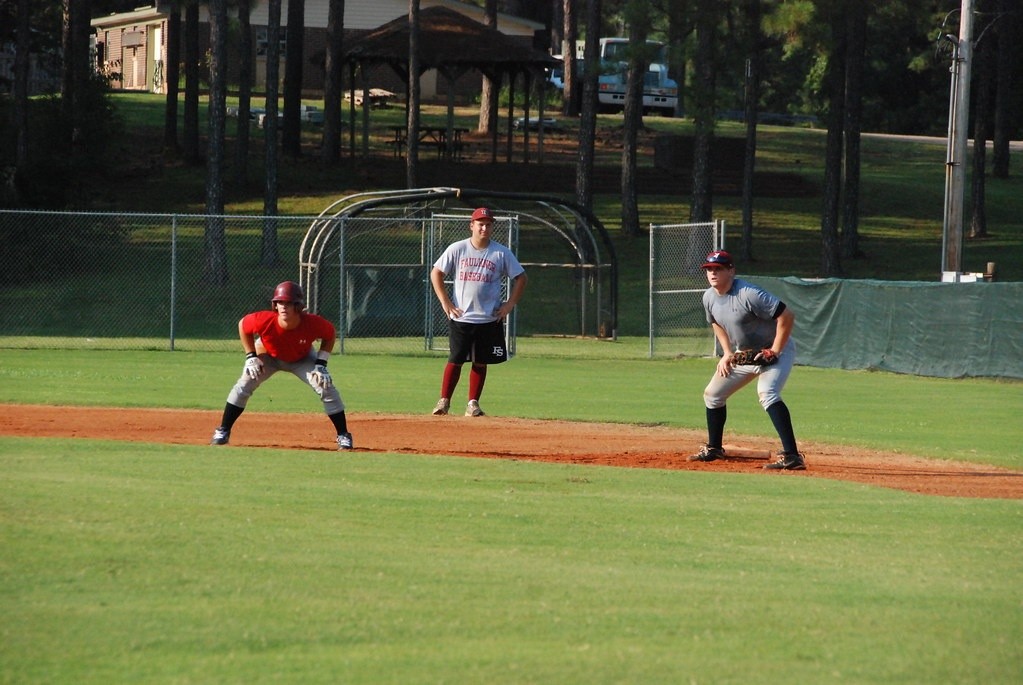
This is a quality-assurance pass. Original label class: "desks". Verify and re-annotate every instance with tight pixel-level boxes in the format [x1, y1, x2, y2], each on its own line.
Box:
[388, 125, 470, 158]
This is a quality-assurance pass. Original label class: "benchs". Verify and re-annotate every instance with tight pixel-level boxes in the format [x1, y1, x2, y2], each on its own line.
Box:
[386, 139, 471, 163]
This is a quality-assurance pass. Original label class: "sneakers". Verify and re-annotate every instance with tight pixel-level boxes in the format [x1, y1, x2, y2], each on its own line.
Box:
[762, 451, 807, 470]
[210, 426, 230, 445]
[337, 432, 352, 451]
[432, 398, 449, 415]
[465, 401, 483, 417]
[685, 442, 726, 461]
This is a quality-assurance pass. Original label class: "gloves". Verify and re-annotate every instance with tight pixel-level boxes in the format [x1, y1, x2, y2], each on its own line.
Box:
[244, 351, 266, 381]
[309, 358, 332, 389]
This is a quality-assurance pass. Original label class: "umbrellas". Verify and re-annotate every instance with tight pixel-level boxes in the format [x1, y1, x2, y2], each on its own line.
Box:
[342, 5, 564, 168]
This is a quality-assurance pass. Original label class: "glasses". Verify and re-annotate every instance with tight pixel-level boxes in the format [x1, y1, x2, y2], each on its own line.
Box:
[700, 256, 733, 267]
[472, 221, 492, 227]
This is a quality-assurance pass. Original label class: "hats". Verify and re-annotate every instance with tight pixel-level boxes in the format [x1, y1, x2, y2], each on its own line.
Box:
[472, 207, 496, 223]
[701, 251, 735, 271]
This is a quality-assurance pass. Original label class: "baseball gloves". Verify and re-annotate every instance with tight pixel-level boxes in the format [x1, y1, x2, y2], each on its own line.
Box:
[729, 348, 777, 367]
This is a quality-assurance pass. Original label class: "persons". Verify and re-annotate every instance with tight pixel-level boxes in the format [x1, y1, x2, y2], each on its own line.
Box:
[686, 249, 806, 471]
[210, 281, 354, 453]
[430, 207, 528, 417]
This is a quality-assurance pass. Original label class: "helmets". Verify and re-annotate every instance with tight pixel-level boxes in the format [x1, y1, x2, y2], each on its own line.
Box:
[271, 281, 305, 314]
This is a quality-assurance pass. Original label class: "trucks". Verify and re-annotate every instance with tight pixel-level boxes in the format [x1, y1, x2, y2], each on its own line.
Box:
[550, 36, 679, 116]
